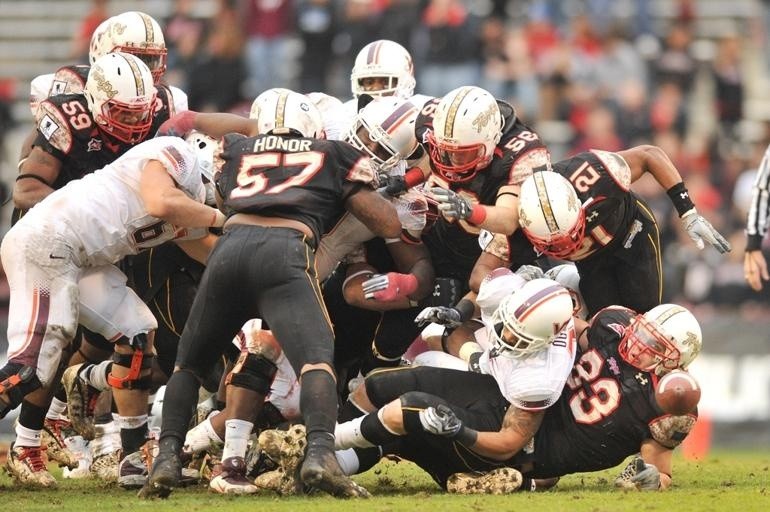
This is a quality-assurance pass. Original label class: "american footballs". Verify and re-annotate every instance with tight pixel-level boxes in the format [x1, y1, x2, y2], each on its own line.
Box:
[657, 371, 700, 415]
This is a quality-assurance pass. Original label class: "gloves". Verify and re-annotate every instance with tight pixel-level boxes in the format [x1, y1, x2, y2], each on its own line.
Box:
[419, 404, 463, 438]
[414, 305, 462, 328]
[515, 264, 544, 280]
[361, 271, 419, 302]
[682, 212, 732, 254]
[430, 186, 473, 220]
[630, 457, 661, 490]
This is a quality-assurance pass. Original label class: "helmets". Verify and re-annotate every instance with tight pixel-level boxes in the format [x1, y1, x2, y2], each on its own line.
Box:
[81, 52, 158, 144]
[350, 39, 416, 97]
[184, 128, 224, 205]
[486, 278, 573, 359]
[518, 171, 587, 259]
[88, 12, 167, 84]
[619, 303, 703, 375]
[250, 86, 505, 182]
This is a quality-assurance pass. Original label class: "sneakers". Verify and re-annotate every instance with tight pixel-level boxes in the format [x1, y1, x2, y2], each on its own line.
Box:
[446, 467, 523, 494]
[2, 416, 374, 499]
[616, 458, 640, 488]
[60, 361, 102, 441]
